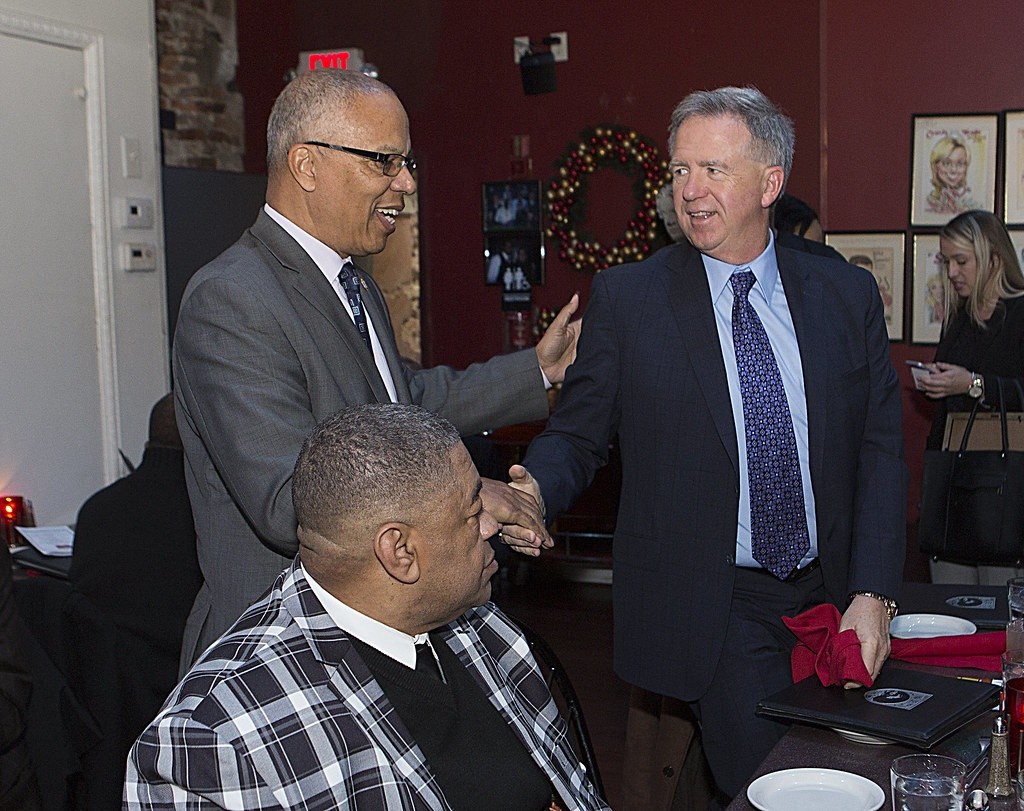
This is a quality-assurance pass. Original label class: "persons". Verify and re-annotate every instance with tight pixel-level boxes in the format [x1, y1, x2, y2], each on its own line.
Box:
[497, 81, 911, 811]
[655, 184, 686, 245]
[486, 237, 537, 282]
[69, 393, 205, 811]
[0, 538, 104, 811]
[174, 63, 582, 688]
[773, 193, 823, 243]
[122, 403, 613, 811]
[488, 185, 538, 225]
[912, 209, 1024, 585]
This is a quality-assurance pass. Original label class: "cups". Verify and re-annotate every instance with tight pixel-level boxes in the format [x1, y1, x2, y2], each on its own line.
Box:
[890, 754, 967, 811]
[1004, 678, 1024, 782]
[1006, 620, 1024, 650]
[1007, 577, 1024, 621]
[1001, 649, 1024, 703]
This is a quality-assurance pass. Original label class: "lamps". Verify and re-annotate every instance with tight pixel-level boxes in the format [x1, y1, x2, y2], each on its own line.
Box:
[283, 47, 379, 84]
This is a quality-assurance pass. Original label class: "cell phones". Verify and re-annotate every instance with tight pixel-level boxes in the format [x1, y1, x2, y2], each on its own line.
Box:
[905, 358, 934, 375]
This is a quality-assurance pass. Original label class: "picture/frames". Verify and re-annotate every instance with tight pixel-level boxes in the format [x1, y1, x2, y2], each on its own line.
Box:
[910, 231, 1024, 347]
[823, 229, 907, 343]
[1001, 108, 1024, 230]
[480, 179, 543, 231]
[481, 231, 546, 285]
[909, 111, 1000, 228]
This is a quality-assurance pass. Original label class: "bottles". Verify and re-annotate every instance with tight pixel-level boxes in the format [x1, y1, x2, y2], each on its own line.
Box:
[984, 715, 1015, 801]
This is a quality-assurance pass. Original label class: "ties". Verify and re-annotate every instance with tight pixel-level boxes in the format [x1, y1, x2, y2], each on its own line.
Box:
[729, 269, 810, 581]
[415, 643, 444, 683]
[338, 262, 374, 361]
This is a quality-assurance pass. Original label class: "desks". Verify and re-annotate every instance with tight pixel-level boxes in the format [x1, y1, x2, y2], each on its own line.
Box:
[727, 629, 1003, 811]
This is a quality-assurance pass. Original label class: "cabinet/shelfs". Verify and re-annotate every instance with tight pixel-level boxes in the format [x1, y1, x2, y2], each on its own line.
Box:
[463, 426, 622, 584]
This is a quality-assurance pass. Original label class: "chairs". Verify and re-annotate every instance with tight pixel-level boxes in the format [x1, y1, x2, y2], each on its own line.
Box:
[504, 615, 607, 808]
[11, 577, 180, 810]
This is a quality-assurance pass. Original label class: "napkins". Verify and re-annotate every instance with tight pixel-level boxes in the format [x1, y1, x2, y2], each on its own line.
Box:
[889, 630, 1008, 671]
[780, 603, 872, 689]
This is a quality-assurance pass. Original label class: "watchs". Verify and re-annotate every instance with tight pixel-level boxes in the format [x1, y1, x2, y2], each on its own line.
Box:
[967, 375, 983, 400]
[849, 592, 898, 621]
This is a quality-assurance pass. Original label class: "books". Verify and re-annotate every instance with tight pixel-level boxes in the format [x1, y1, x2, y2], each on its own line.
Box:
[755, 665, 1003, 753]
[898, 583, 1011, 631]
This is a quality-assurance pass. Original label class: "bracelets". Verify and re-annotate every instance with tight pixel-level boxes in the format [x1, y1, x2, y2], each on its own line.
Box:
[966, 372, 975, 394]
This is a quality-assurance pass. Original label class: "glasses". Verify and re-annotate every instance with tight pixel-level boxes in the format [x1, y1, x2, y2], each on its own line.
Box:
[287, 141, 415, 178]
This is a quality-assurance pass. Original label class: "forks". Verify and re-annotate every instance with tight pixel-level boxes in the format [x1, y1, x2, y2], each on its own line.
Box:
[966, 734, 991, 776]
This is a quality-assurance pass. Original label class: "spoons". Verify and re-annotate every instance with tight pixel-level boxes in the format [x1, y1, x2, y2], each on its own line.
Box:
[964, 789, 989, 811]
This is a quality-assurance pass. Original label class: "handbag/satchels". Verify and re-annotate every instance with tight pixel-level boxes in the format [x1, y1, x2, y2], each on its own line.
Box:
[918, 377, 1024, 567]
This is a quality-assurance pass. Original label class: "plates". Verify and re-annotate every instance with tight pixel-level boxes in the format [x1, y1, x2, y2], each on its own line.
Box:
[831, 727, 897, 745]
[747, 768, 885, 811]
[889, 614, 977, 639]
